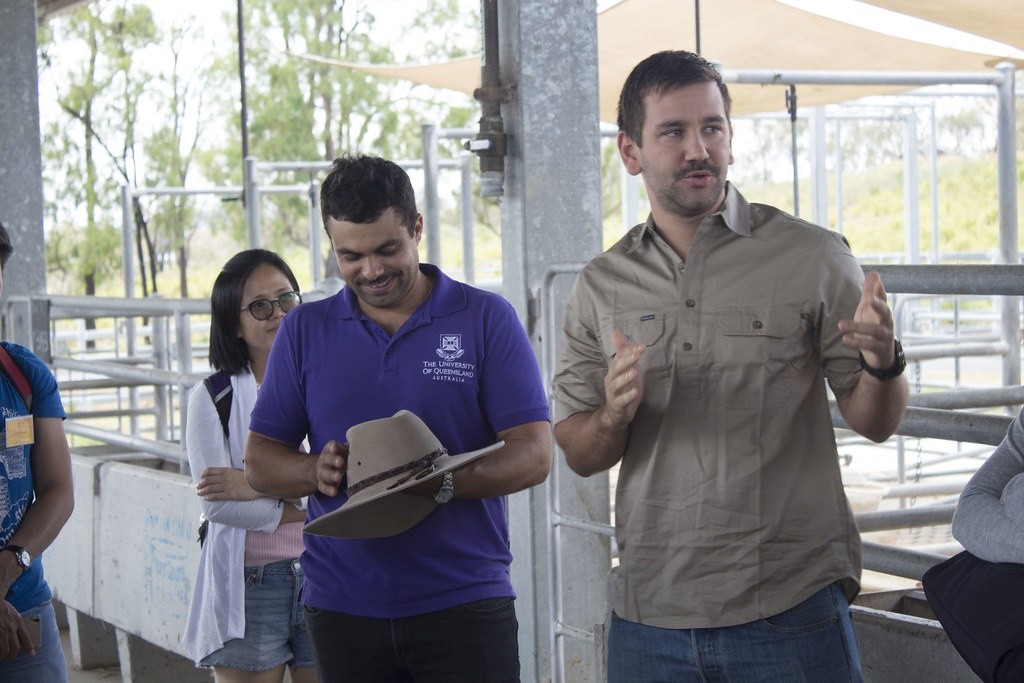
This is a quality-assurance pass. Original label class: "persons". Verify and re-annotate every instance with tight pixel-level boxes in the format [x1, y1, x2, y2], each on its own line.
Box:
[244, 155, 553, 683]
[552, 51, 909, 683]
[951, 407, 1024, 563]
[0, 225, 74, 683]
[182, 250, 319, 683]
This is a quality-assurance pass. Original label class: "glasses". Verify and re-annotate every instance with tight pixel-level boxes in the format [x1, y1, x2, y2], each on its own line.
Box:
[237, 291, 303, 322]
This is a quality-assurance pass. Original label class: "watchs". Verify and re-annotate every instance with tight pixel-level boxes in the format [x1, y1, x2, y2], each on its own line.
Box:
[859, 340, 907, 380]
[433, 471, 454, 505]
[2, 544, 31, 578]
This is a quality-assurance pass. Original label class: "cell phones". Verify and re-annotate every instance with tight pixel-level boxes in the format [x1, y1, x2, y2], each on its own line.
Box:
[22, 614, 41, 649]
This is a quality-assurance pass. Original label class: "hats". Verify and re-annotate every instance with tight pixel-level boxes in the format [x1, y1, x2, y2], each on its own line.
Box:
[300, 409, 505, 539]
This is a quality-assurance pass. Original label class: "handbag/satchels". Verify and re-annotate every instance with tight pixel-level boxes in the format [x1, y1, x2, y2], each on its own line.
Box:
[186, 369, 234, 551]
[922, 550, 1024, 683]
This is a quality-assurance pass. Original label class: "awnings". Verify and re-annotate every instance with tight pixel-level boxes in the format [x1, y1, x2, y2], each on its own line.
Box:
[292, 0, 1024, 124]
